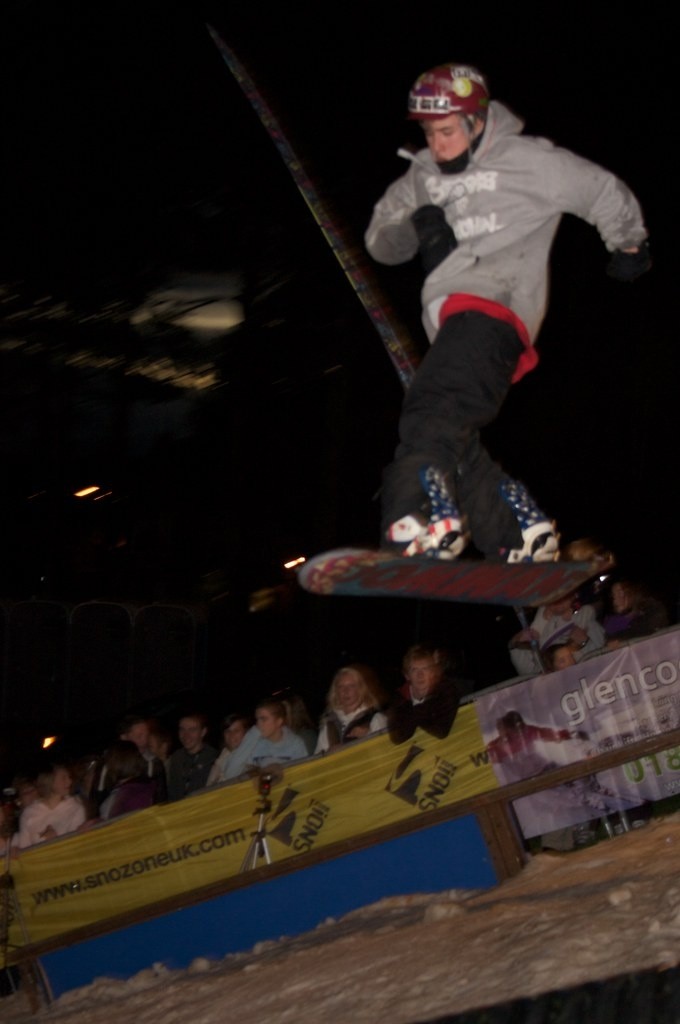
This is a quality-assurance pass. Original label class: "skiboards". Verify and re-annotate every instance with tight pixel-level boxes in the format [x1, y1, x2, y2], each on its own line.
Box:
[576, 784, 656, 815]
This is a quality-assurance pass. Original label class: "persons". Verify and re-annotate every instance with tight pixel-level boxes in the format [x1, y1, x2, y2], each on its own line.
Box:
[0, 580, 669, 853]
[364, 64, 645, 565]
[490, 710, 593, 802]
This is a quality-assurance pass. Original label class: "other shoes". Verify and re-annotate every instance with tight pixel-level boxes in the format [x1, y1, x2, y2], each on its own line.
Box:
[632, 819, 645, 828]
[613, 824, 623, 835]
[387, 514, 467, 561]
[577, 832, 590, 844]
[496, 522, 557, 564]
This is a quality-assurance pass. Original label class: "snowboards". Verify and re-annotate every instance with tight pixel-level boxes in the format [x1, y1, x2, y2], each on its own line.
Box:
[298, 545, 601, 608]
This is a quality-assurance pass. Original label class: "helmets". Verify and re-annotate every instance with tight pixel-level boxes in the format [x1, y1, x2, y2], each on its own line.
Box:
[408, 65, 487, 122]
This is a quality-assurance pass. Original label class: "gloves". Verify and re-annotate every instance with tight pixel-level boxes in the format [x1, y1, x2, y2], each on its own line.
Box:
[410, 205, 445, 247]
